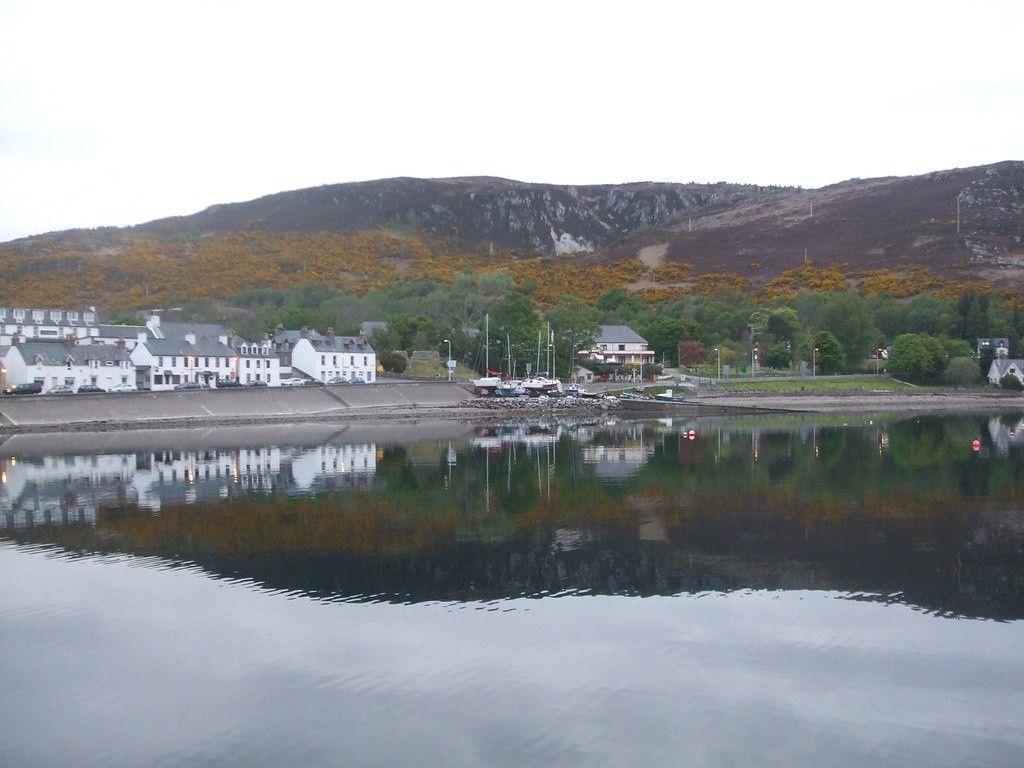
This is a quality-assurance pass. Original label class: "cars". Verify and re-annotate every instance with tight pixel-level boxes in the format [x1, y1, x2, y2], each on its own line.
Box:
[174, 381, 200, 390]
[346, 376, 366, 384]
[281, 377, 304, 386]
[304, 377, 326, 387]
[249, 379, 268, 386]
[47, 385, 74, 394]
[78, 384, 106, 393]
[327, 376, 347, 384]
[108, 382, 138, 392]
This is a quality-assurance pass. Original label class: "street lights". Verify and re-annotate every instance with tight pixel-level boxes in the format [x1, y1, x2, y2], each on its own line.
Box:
[548, 343, 556, 380]
[751, 348, 758, 378]
[812, 348, 818, 377]
[876, 348, 881, 376]
[444, 339, 451, 382]
[714, 348, 721, 380]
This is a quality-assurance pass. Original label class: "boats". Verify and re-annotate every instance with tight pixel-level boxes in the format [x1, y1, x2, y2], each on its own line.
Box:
[655, 389, 686, 403]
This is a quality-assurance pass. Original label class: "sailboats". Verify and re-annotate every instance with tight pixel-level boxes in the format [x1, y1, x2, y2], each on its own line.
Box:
[470, 424, 563, 512]
[469, 313, 564, 397]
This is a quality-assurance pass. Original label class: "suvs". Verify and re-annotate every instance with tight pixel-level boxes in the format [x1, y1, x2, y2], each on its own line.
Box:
[2, 382, 42, 396]
[216, 379, 243, 388]
